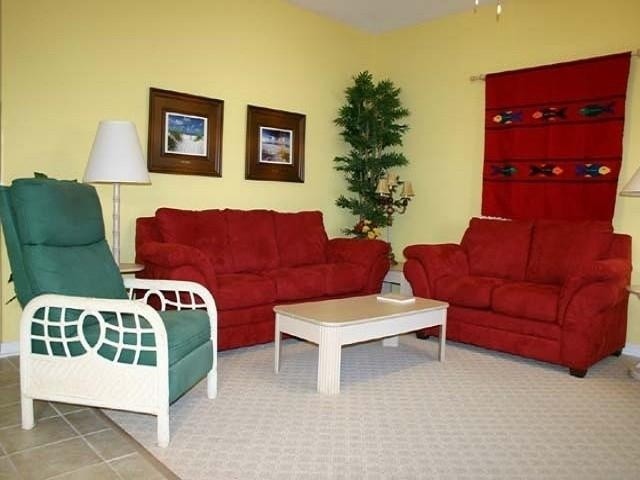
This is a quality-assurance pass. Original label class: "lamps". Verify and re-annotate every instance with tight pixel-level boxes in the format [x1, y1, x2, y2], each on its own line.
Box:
[82, 118, 153, 274]
[619, 166, 640, 197]
[472, 0, 504, 23]
[373, 176, 416, 268]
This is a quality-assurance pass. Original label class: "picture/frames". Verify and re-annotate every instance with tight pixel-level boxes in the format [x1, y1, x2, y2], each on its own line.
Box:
[245, 103, 308, 185]
[147, 85, 224, 178]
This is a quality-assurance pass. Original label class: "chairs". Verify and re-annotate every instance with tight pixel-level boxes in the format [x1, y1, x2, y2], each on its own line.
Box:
[1, 178, 218, 448]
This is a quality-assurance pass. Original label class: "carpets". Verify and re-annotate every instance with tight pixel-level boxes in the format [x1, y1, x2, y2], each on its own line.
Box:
[98, 319, 640, 479]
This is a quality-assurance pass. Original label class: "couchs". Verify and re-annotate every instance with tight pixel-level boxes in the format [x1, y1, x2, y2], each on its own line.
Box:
[135, 208, 396, 349]
[403, 215, 635, 379]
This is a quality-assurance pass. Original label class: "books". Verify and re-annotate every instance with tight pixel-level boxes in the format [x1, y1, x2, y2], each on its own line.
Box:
[376, 292, 415, 304]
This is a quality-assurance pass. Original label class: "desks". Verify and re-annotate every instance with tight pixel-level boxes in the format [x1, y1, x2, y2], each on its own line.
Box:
[624, 285, 640, 381]
[380, 262, 414, 296]
[114, 263, 145, 272]
